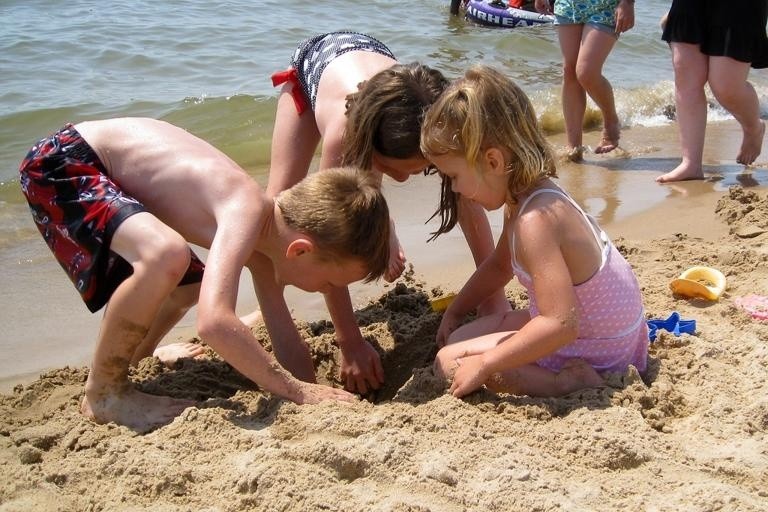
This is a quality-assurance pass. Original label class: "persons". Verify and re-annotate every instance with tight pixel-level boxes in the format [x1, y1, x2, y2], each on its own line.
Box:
[237, 31, 513, 329]
[19, 116, 389, 434]
[420, 66, 648, 397]
[535, 0, 634, 158]
[655, 0, 768, 182]
